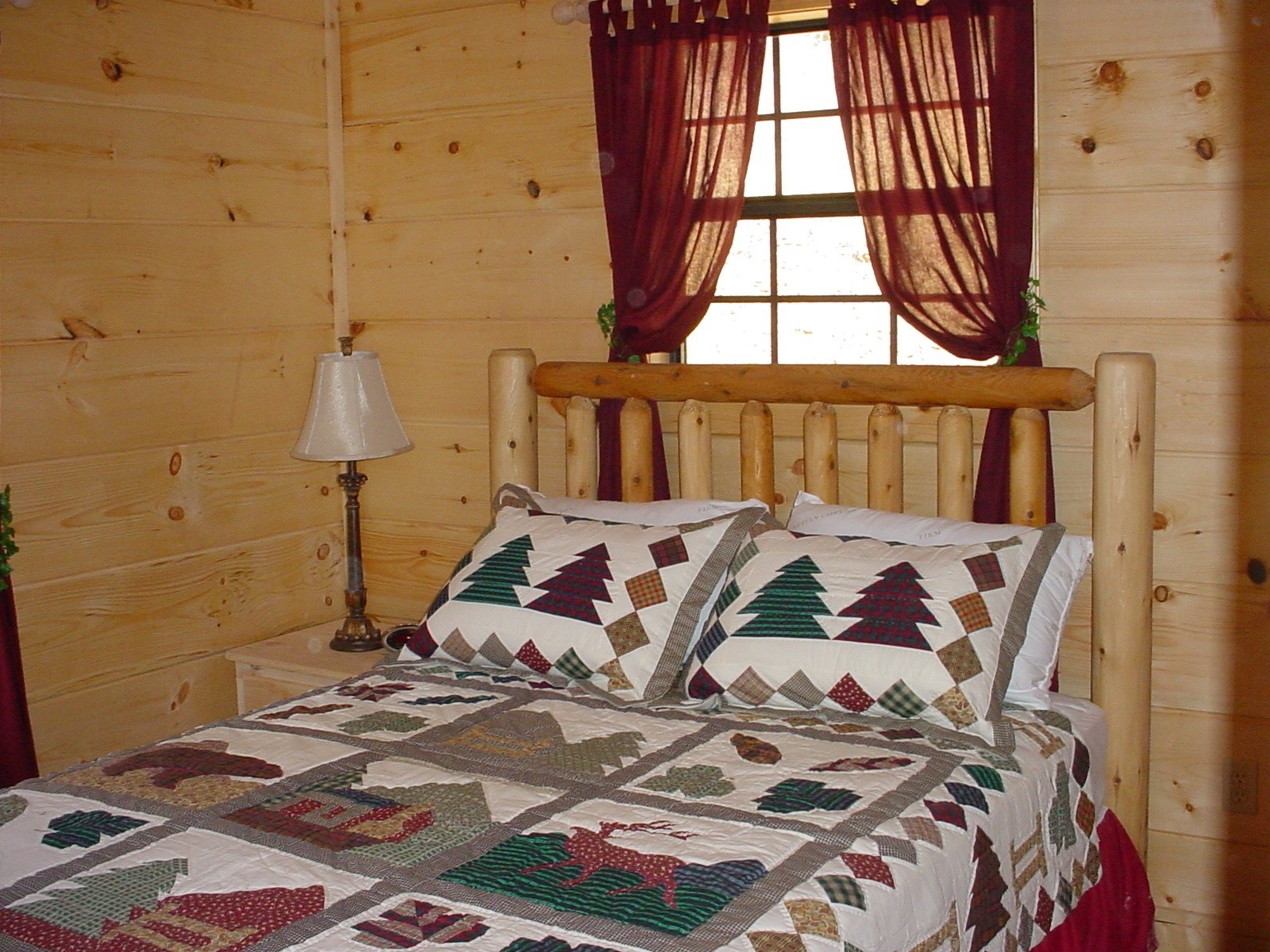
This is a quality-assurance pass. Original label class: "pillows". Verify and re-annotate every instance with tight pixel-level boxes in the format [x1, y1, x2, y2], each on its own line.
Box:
[680, 503, 1068, 763]
[392, 479, 766, 710]
[519, 480, 758, 670]
[785, 488, 1097, 716]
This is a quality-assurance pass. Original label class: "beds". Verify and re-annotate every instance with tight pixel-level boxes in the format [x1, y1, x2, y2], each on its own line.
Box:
[2, 345, 1168, 952]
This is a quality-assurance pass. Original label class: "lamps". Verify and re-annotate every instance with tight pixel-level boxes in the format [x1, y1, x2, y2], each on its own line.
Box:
[291, 337, 415, 655]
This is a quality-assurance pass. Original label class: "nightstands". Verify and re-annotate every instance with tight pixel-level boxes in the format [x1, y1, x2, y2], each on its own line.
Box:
[222, 615, 414, 717]
